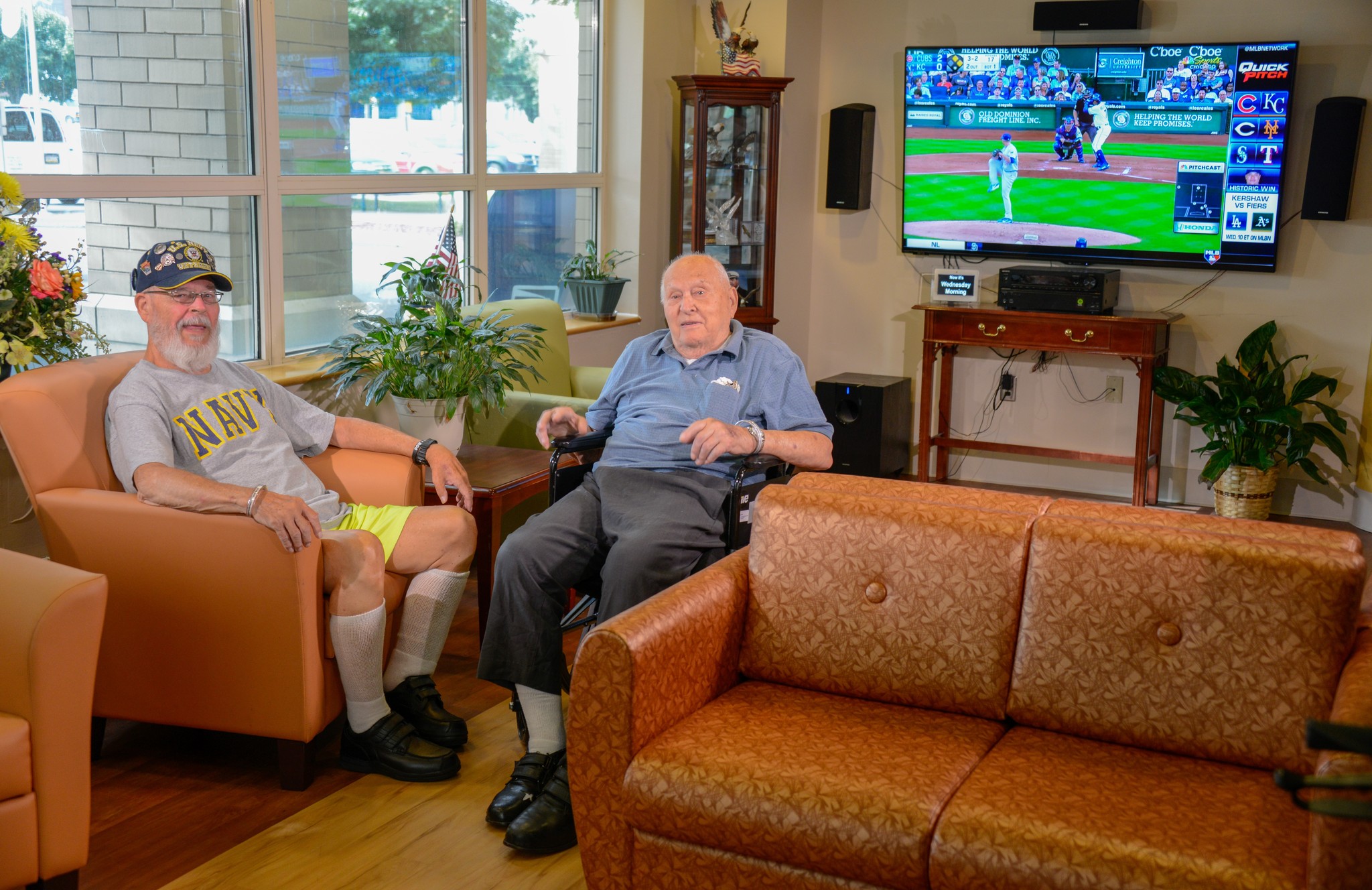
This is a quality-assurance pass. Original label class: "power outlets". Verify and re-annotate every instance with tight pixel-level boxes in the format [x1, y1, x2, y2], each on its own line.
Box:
[999, 376, 1017, 401]
[1105, 376, 1123, 403]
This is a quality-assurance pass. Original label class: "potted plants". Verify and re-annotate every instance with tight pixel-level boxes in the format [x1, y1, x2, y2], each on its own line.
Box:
[1153, 319, 1352, 522]
[306, 255, 553, 460]
[558, 240, 645, 321]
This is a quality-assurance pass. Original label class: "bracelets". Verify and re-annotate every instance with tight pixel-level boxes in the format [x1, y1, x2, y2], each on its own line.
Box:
[734, 420, 762, 454]
[412, 441, 425, 465]
[246, 484, 268, 518]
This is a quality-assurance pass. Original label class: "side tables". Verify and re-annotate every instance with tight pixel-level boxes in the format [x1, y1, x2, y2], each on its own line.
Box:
[416, 440, 579, 655]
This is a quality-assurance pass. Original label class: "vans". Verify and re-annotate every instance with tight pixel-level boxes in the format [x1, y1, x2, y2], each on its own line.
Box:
[0, 104, 83, 204]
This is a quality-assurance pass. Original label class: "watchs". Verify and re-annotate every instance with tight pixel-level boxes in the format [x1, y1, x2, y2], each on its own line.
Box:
[416, 438, 438, 467]
[745, 425, 765, 454]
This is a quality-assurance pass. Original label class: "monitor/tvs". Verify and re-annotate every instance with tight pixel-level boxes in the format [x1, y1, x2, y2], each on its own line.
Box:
[903, 41, 1298, 274]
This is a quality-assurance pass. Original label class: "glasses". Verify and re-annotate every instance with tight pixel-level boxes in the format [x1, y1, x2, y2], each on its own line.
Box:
[1015, 89, 1021, 92]
[143, 290, 224, 304]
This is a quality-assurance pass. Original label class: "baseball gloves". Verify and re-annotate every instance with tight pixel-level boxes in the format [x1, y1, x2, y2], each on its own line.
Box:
[992, 149, 1003, 161]
[1062, 140, 1075, 149]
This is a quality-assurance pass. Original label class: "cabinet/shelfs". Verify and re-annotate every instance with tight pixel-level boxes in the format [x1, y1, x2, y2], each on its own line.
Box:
[670, 72, 795, 331]
[911, 300, 1185, 507]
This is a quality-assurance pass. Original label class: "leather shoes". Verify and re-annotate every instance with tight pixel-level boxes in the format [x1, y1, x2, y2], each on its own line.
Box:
[384, 674, 469, 747]
[502, 756, 577, 853]
[338, 711, 461, 782]
[485, 748, 566, 828]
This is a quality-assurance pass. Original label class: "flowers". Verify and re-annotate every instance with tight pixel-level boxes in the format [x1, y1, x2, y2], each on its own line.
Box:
[0, 171, 111, 372]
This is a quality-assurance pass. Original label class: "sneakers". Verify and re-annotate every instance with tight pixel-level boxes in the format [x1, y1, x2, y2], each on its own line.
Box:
[1092, 162, 1110, 171]
[997, 217, 1012, 223]
[987, 182, 1000, 193]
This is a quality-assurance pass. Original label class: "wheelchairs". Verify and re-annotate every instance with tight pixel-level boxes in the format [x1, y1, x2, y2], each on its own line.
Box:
[491, 423, 796, 750]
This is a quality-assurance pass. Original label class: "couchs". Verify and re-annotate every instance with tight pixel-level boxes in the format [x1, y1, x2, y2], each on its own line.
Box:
[0, 547, 108, 890]
[0, 350, 426, 788]
[567, 472, 1372, 890]
[413, 298, 614, 449]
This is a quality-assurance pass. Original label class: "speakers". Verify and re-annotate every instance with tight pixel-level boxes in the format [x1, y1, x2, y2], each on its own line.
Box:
[825, 103, 876, 210]
[1300, 96, 1369, 220]
[813, 373, 910, 474]
[1032, 0, 1144, 30]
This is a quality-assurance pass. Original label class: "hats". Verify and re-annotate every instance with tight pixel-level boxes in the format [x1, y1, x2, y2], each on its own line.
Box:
[1000, 133, 1011, 141]
[1014, 87, 1022, 92]
[1014, 55, 1020, 59]
[1172, 87, 1180, 94]
[1033, 57, 1041, 62]
[131, 239, 233, 294]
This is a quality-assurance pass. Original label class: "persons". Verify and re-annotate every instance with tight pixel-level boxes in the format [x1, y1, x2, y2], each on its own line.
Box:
[906, 54, 1234, 223]
[104, 240, 478, 782]
[476, 250, 834, 850]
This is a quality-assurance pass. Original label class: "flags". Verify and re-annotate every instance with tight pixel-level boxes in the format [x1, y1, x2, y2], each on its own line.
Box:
[427, 215, 461, 307]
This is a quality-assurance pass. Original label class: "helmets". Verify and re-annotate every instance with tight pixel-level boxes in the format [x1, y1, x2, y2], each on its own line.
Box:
[1208, 66, 1217, 72]
[1082, 89, 1091, 96]
[1092, 93, 1101, 103]
[1064, 116, 1074, 125]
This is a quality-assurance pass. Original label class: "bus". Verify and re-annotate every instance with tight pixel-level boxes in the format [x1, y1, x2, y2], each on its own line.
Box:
[347, 51, 539, 195]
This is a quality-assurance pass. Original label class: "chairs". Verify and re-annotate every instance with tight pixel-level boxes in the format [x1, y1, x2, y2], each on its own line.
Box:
[1148, 73, 1229, 102]
[908, 70, 1094, 101]
